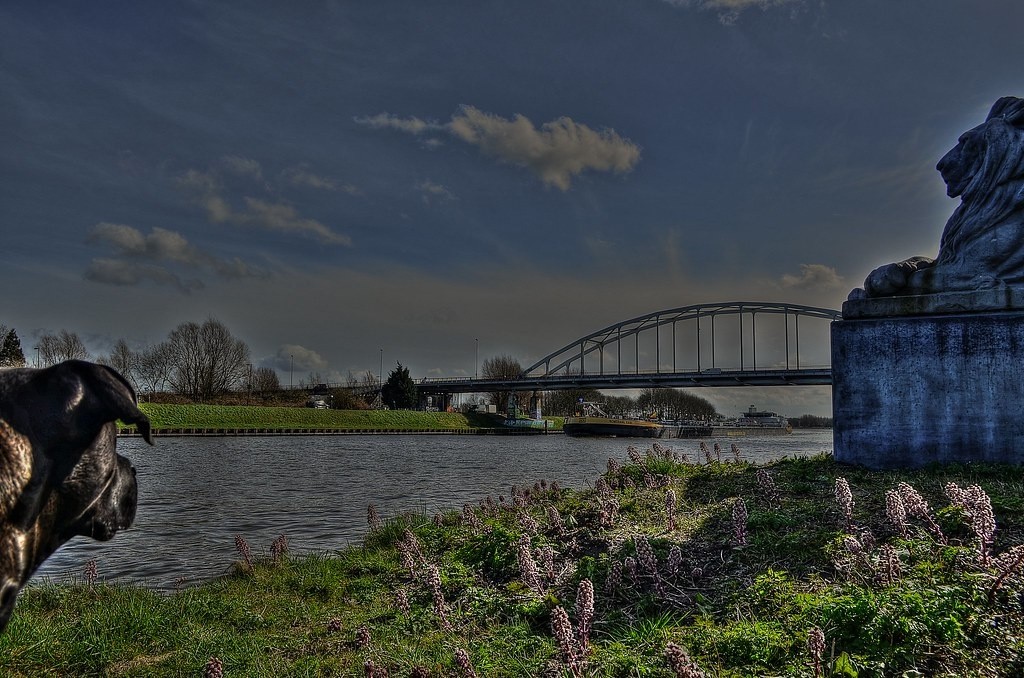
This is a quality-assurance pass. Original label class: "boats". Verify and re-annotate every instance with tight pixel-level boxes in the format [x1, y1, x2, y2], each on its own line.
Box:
[562, 415, 667, 439]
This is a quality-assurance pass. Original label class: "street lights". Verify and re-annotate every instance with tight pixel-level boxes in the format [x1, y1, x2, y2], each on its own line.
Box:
[474, 337, 479, 381]
[290, 353, 294, 393]
[379, 348, 384, 390]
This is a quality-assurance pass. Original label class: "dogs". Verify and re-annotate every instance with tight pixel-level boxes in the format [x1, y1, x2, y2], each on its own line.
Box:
[0, 359, 155, 639]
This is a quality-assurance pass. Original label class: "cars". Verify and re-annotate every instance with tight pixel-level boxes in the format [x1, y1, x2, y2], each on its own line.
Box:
[305, 400, 330, 409]
